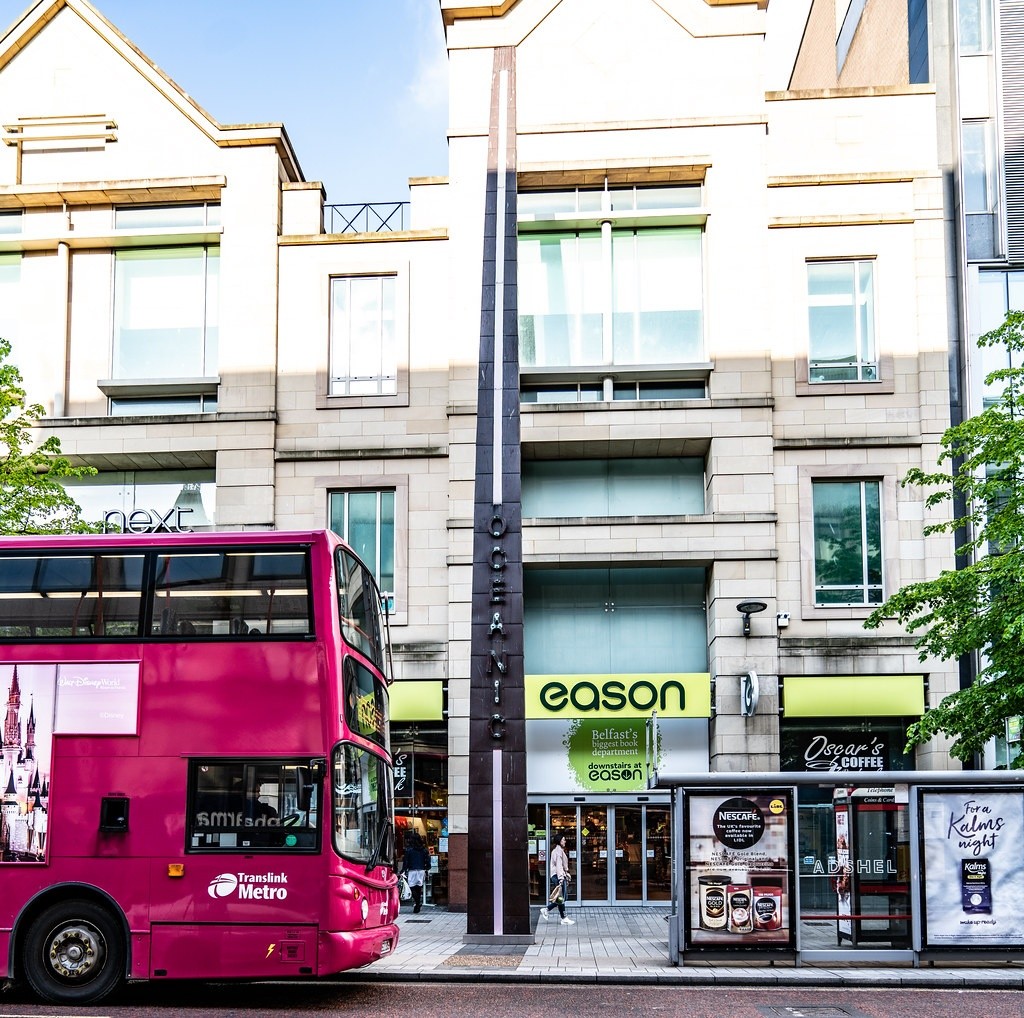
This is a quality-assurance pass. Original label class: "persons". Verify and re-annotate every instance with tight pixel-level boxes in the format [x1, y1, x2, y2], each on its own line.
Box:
[539, 833, 575, 926]
[239, 778, 277, 820]
[394, 833, 398, 862]
[398, 832, 431, 913]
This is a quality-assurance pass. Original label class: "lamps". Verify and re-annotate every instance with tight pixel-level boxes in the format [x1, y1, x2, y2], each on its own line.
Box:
[735, 600, 767, 636]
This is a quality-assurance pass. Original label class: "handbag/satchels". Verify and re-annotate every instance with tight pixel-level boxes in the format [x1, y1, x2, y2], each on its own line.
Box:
[548, 884, 565, 905]
[400, 877, 412, 901]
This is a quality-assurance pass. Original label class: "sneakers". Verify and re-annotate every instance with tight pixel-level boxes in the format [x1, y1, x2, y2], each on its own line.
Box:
[540, 907, 549, 920]
[560, 917, 574, 925]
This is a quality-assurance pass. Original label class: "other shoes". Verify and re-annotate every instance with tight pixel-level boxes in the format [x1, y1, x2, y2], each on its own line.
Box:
[413, 906, 420, 913]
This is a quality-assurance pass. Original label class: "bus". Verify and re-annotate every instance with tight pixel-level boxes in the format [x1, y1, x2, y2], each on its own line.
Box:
[1, 526, 400, 1005]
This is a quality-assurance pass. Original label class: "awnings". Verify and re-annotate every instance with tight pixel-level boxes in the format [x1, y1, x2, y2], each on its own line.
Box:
[388, 681, 443, 722]
[783, 676, 925, 716]
